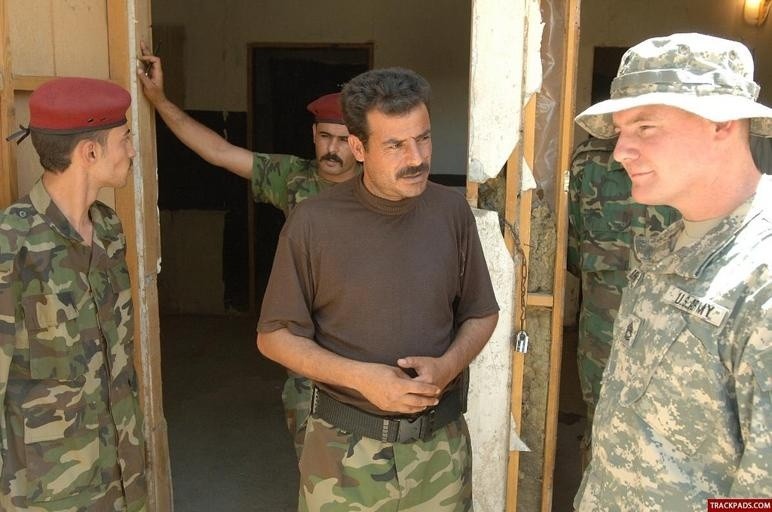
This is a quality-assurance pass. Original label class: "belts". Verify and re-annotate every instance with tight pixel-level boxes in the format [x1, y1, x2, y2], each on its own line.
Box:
[310, 385, 462, 444]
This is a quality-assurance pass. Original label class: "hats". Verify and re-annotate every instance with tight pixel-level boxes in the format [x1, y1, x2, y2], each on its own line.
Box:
[25, 76, 131, 136]
[575, 31, 772, 140]
[307, 93, 347, 125]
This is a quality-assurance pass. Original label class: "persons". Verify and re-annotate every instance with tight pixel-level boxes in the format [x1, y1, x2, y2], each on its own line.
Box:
[256, 63, 501, 512]
[563, 111, 679, 484]
[571, 30, 771, 512]
[1, 74, 149, 509]
[136, 42, 364, 463]
